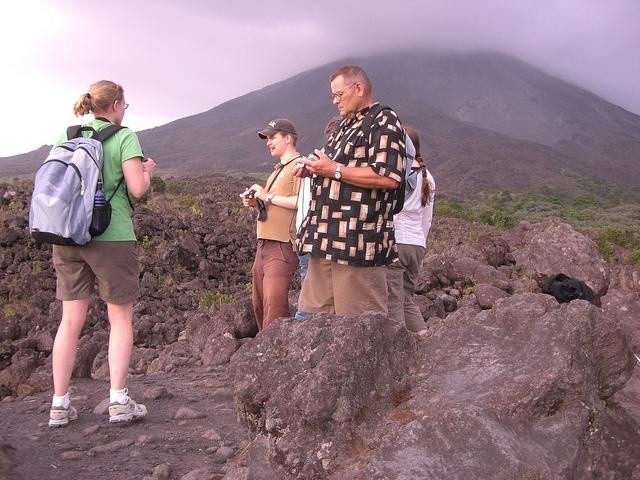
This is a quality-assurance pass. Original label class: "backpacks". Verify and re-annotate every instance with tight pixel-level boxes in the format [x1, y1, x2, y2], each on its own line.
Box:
[364, 106, 417, 214]
[29, 125, 129, 245]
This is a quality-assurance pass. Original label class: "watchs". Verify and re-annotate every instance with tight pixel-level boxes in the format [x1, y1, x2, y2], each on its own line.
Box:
[268, 194, 274, 204]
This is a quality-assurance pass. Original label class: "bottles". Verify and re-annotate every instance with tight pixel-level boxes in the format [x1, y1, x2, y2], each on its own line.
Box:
[90, 178, 108, 231]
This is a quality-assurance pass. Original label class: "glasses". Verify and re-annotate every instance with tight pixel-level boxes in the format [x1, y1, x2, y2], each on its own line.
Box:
[329, 84, 356, 100]
[122, 103, 129, 109]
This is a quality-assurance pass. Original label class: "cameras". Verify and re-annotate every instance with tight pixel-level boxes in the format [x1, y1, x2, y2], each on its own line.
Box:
[305, 154, 318, 167]
[243, 189, 256, 200]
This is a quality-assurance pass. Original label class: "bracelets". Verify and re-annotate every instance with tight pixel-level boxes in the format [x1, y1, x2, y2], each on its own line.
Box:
[144, 169, 152, 176]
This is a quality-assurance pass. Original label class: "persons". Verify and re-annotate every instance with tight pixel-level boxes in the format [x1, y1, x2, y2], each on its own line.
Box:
[240, 119, 306, 330]
[49, 80, 155, 428]
[291, 65, 436, 335]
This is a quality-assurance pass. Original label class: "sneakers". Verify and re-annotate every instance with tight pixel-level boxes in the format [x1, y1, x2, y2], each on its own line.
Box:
[48, 401, 78, 427]
[109, 398, 147, 424]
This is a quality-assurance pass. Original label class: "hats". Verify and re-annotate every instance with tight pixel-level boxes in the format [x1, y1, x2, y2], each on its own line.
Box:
[258, 118, 297, 139]
